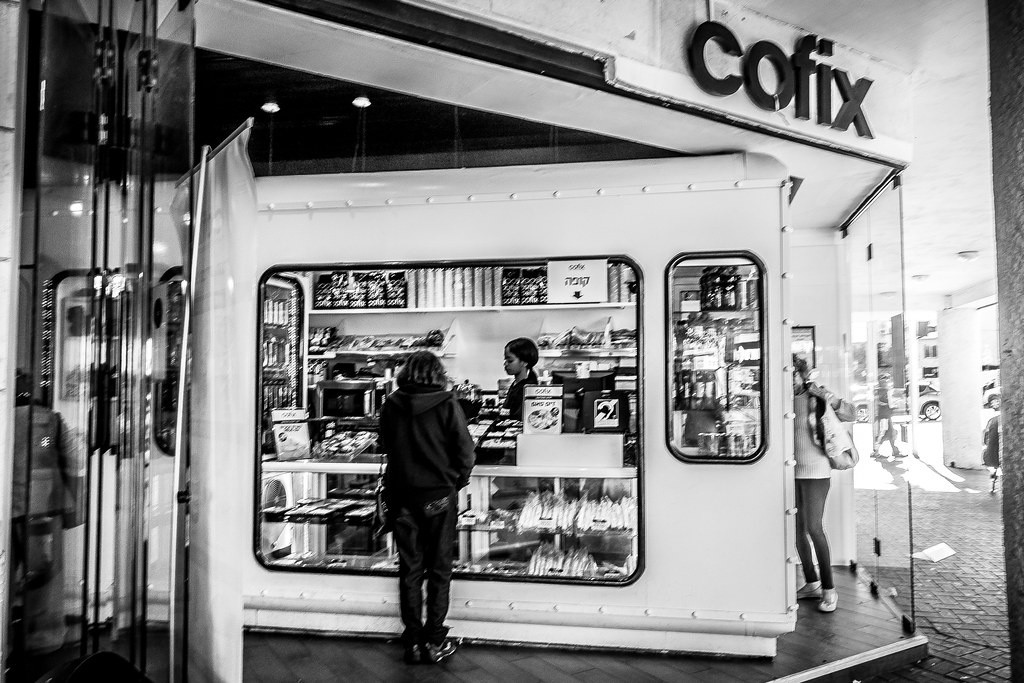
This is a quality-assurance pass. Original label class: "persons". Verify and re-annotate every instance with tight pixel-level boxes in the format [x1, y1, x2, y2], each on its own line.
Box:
[13, 368, 87, 649]
[870, 373, 908, 458]
[981, 414, 1001, 466]
[793, 353, 859, 610]
[374, 352, 476, 663]
[497, 335, 538, 422]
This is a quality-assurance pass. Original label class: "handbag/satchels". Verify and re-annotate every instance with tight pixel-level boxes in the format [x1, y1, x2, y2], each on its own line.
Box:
[47, 413, 86, 529]
[809, 385, 859, 470]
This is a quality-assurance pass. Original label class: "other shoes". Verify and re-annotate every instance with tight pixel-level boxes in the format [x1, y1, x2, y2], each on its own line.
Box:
[425, 638, 456, 662]
[404, 644, 422, 664]
[797, 581, 823, 598]
[870, 453, 888, 458]
[818, 588, 838, 611]
[895, 454, 908, 457]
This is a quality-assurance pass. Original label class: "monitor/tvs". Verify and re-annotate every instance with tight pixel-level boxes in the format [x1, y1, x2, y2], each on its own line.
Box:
[553, 370, 616, 433]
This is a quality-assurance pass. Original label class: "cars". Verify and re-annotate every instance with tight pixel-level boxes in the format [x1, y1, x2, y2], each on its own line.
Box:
[849, 382, 941, 424]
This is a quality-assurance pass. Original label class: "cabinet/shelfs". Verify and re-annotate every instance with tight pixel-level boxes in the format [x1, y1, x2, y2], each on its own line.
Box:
[307, 262, 637, 422]
[671, 259, 763, 457]
[262, 453, 637, 580]
